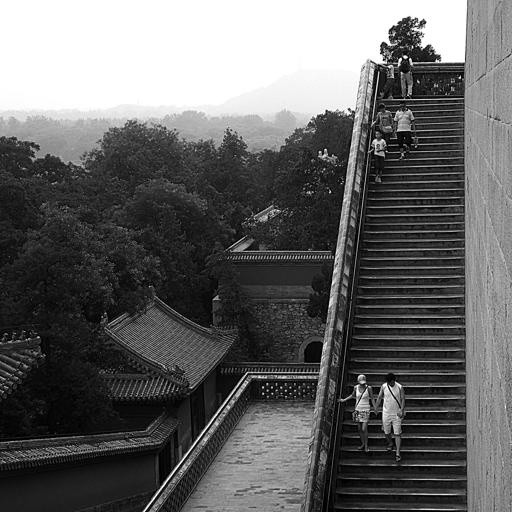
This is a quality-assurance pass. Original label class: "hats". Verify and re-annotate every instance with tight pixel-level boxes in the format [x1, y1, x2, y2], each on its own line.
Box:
[357, 374, 367, 386]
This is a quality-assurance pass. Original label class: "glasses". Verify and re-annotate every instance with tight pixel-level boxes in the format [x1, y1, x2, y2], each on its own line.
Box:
[400, 105, 406, 107]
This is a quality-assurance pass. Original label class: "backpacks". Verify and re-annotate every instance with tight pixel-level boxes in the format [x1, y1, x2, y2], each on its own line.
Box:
[400, 56, 411, 73]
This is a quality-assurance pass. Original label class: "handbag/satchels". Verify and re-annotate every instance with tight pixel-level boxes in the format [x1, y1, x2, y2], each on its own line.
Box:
[351, 410, 360, 423]
[414, 134, 420, 149]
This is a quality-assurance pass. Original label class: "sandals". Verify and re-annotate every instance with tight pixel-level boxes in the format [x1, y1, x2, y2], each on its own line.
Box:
[395, 455, 402, 463]
[386, 445, 394, 452]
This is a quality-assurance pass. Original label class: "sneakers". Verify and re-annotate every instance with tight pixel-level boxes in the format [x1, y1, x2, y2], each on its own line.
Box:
[399, 152, 405, 160]
[357, 444, 370, 454]
[388, 95, 394, 99]
[400, 94, 412, 99]
[375, 175, 382, 183]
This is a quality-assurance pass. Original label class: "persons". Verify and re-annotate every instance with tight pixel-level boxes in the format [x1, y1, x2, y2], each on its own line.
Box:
[398, 50, 414, 99]
[370, 102, 395, 151]
[377, 59, 395, 99]
[337, 374, 378, 454]
[367, 129, 388, 183]
[374, 373, 406, 462]
[393, 101, 417, 159]
